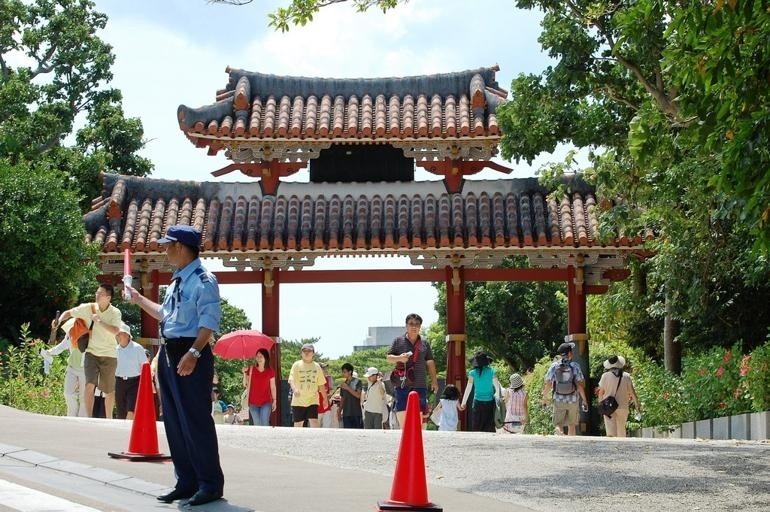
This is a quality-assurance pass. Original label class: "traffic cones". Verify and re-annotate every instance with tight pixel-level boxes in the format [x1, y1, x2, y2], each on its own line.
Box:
[375, 390, 448, 512]
[109, 360, 176, 461]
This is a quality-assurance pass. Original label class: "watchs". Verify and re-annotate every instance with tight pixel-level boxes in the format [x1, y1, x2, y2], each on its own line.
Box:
[189, 346, 201, 359]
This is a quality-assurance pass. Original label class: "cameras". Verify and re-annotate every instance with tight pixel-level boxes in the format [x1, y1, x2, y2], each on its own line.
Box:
[406, 351, 413, 357]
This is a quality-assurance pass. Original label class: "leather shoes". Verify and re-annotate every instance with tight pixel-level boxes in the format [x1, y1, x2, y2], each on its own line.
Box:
[157, 486, 196, 501]
[184, 489, 223, 507]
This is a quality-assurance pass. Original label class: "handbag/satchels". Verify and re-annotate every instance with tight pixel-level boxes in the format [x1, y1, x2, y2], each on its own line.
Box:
[597, 395, 619, 418]
[428, 407, 442, 425]
[495, 396, 507, 430]
[389, 356, 419, 389]
[239, 386, 251, 411]
[68, 317, 92, 354]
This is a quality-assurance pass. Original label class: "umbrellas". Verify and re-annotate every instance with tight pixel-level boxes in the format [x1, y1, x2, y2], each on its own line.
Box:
[212, 329, 275, 374]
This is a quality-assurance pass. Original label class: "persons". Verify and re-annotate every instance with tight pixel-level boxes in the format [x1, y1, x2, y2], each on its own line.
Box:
[432, 384, 466, 431]
[504, 374, 528, 433]
[541, 341, 588, 436]
[387, 313, 439, 429]
[41, 284, 164, 421]
[461, 351, 505, 431]
[120, 224, 223, 506]
[211, 344, 445, 429]
[597, 354, 641, 436]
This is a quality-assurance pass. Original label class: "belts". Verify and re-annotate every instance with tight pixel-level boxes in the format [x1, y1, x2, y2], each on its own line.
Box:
[159, 336, 196, 346]
[115, 375, 141, 382]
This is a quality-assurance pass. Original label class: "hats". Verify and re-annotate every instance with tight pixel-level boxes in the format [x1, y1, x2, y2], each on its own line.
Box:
[363, 366, 379, 378]
[558, 341, 576, 356]
[603, 355, 626, 370]
[302, 343, 316, 354]
[119, 323, 134, 340]
[509, 373, 527, 390]
[471, 351, 492, 367]
[318, 361, 330, 368]
[157, 225, 204, 248]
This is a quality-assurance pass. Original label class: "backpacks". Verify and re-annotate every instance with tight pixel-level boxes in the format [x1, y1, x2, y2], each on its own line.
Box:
[554, 360, 577, 396]
